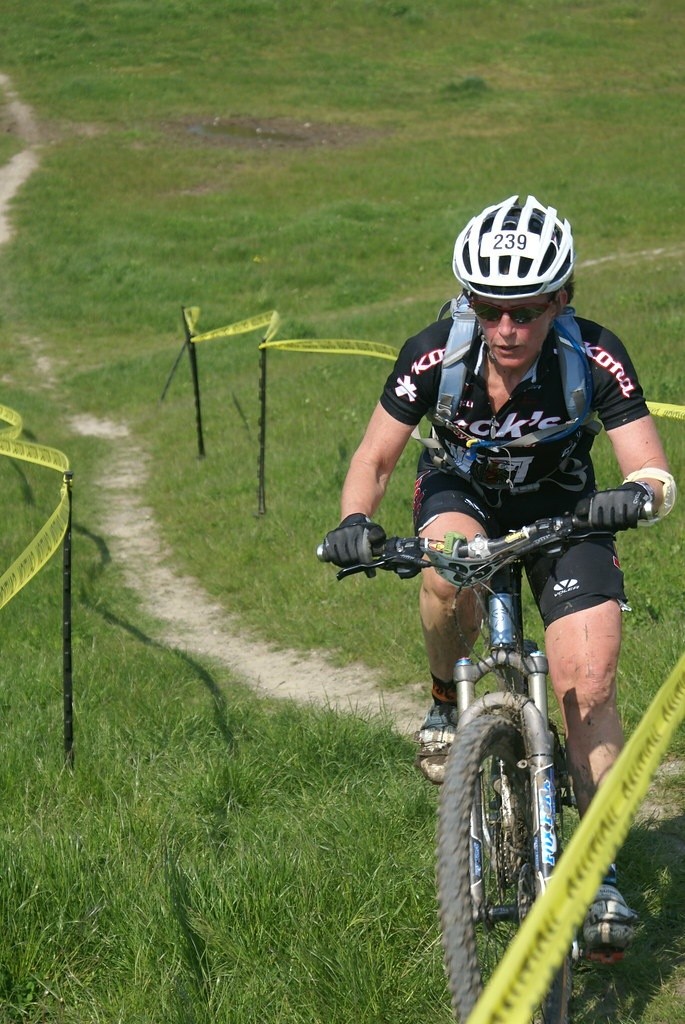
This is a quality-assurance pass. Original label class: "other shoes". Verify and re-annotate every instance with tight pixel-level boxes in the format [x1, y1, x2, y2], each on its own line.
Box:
[413, 703, 458, 786]
[582, 885, 638, 950]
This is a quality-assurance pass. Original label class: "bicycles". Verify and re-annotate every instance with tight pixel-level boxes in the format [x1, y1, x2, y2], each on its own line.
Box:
[317, 500, 657, 1023]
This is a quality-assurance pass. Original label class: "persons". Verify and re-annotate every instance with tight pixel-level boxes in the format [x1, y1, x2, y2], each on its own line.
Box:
[320, 196, 676, 950]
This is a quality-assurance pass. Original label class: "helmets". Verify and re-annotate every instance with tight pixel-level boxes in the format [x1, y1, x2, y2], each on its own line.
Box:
[452, 192, 577, 302]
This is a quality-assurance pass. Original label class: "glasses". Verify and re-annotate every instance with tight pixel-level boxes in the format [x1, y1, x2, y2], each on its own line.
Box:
[462, 291, 555, 325]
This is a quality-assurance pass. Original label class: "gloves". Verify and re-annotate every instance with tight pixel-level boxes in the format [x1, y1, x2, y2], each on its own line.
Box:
[322, 512, 387, 579]
[569, 478, 657, 534]
